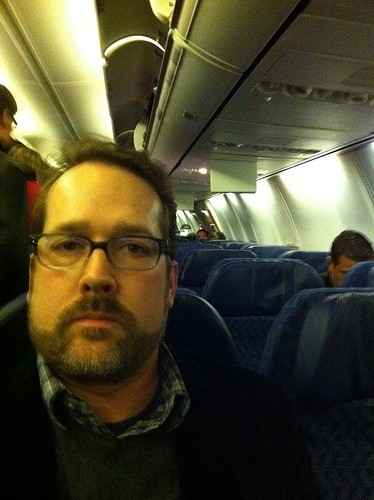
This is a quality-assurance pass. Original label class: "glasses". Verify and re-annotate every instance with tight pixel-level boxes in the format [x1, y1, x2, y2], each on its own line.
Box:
[6, 113, 18, 131]
[29, 233, 174, 271]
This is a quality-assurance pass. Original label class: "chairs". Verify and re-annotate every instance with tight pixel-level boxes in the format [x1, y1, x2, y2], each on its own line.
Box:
[0, 237, 374, 500]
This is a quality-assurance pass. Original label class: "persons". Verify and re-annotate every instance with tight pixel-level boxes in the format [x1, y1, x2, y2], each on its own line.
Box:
[320, 230, 374, 288]
[0, 84, 53, 310]
[0, 134, 295, 500]
[197, 229, 209, 237]
